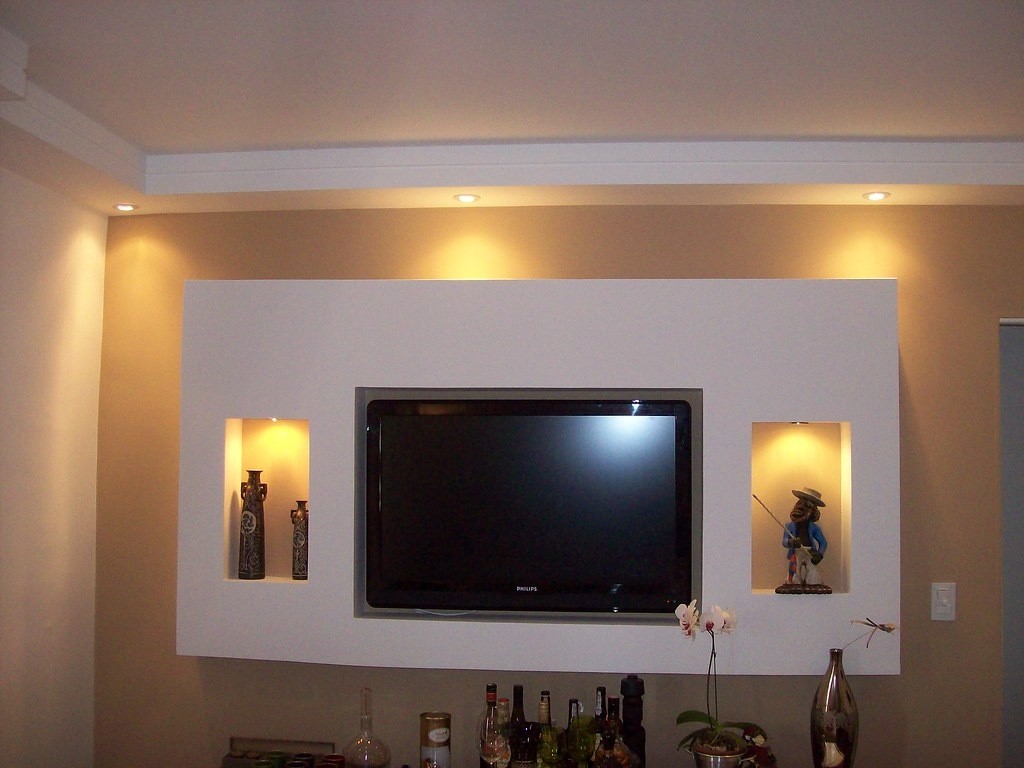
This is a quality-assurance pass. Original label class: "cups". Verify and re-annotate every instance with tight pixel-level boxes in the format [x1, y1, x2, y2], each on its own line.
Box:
[315, 754, 346, 768]
[565, 714, 596, 761]
[474, 712, 508, 764]
[538, 724, 564, 763]
[253, 750, 288, 768]
[285, 752, 315, 768]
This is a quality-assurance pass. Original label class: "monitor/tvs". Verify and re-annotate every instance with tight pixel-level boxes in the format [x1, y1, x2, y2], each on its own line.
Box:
[355, 386, 706, 626]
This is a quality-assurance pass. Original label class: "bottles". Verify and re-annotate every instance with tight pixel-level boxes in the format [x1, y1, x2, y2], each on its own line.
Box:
[480, 682, 623, 768]
[343, 687, 392, 768]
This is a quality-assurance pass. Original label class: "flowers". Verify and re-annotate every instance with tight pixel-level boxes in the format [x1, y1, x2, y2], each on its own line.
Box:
[675, 599, 768, 752]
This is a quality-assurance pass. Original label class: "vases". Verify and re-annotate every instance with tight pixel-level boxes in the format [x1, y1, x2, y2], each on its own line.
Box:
[693, 743, 745, 768]
